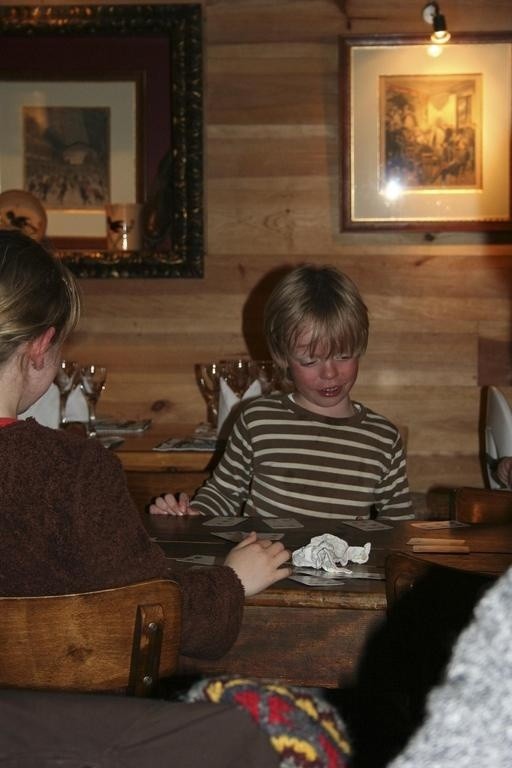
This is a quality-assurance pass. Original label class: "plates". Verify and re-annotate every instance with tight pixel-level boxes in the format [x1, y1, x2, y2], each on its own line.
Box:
[1, 190, 49, 242]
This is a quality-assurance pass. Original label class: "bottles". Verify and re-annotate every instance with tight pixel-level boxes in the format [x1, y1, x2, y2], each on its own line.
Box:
[103, 201, 142, 250]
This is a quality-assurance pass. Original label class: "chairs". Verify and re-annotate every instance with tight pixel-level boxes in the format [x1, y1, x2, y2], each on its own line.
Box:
[448, 487, 511, 525]
[1, 577, 185, 697]
[384, 550, 503, 609]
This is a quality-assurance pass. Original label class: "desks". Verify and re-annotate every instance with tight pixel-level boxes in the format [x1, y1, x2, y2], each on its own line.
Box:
[137, 512, 512, 689]
[63, 423, 409, 504]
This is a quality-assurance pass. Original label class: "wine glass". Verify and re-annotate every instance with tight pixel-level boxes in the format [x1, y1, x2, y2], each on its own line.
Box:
[74, 364, 109, 442]
[46, 360, 82, 430]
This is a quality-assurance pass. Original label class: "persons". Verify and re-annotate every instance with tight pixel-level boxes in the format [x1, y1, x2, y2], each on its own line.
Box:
[496, 457, 512, 491]
[0, 227, 294, 703]
[28, 126, 108, 210]
[150, 264, 417, 521]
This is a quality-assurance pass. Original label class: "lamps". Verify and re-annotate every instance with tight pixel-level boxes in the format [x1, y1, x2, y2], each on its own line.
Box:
[422, 0, 452, 44]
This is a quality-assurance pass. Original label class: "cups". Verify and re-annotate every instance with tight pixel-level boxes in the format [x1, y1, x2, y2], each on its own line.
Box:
[248, 358, 279, 395]
[190, 361, 222, 429]
[213, 356, 257, 398]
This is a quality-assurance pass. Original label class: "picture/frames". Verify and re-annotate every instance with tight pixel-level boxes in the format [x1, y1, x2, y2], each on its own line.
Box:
[339, 30, 512, 232]
[0, 0, 204, 280]
